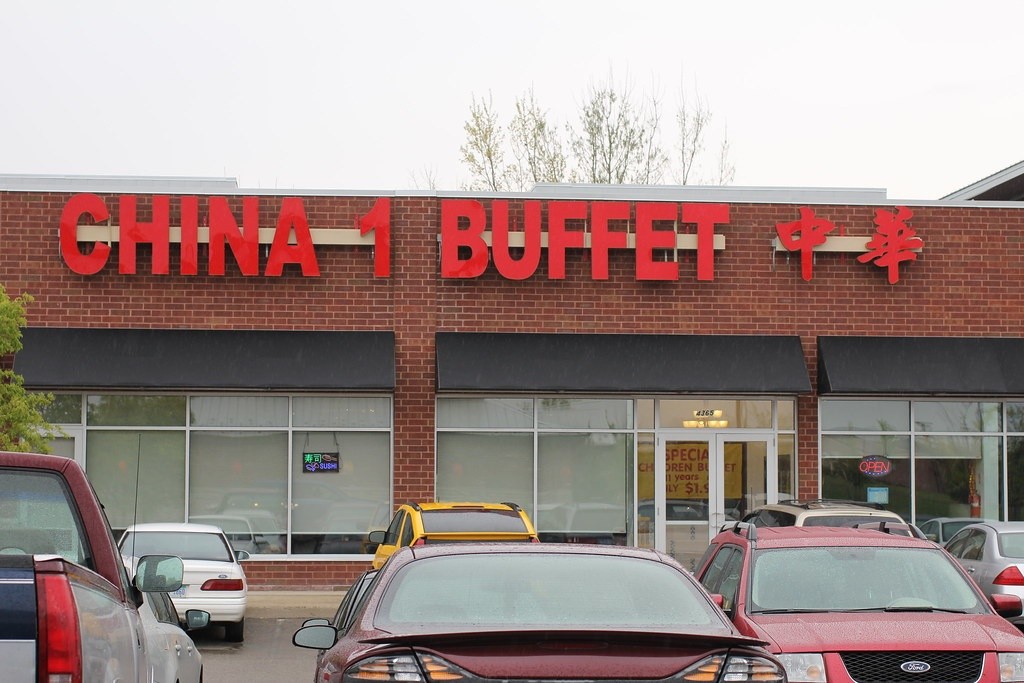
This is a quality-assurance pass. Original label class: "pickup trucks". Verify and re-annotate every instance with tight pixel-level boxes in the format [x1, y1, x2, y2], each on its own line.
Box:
[0, 452, 207, 682]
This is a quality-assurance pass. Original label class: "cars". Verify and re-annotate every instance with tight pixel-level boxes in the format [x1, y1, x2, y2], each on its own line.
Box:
[368, 504, 537, 570]
[694, 530, 1023, 682]
[117, 521, 240, 643]
[291, 545, 789, 682]
[736, 499, 918, 548]
[945, 522, 1024, 618]
[183, 487, 990, 575]
